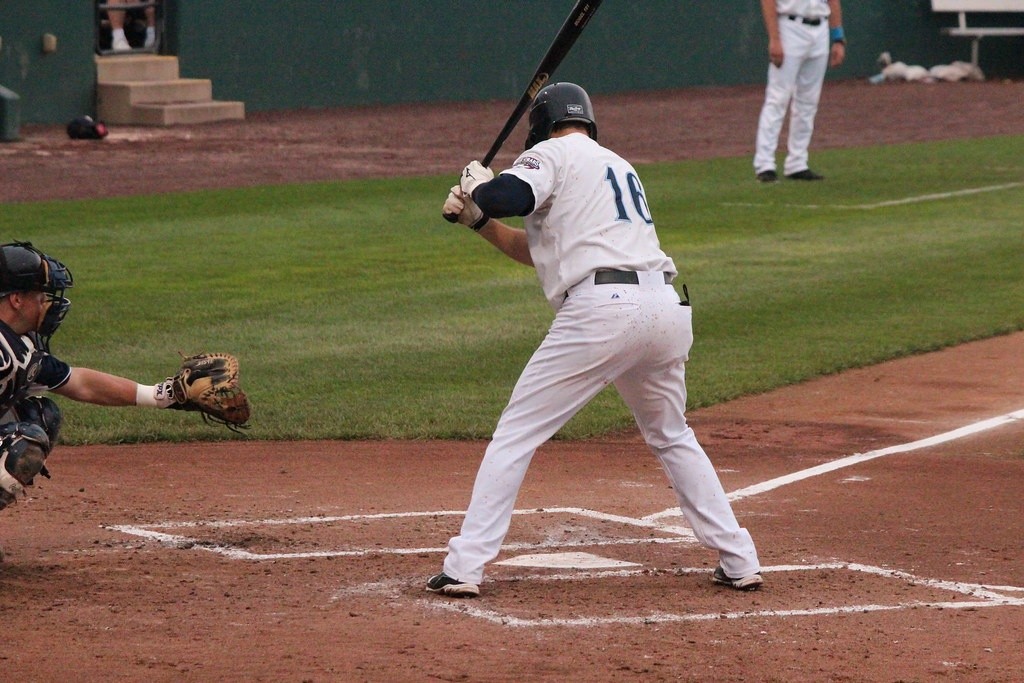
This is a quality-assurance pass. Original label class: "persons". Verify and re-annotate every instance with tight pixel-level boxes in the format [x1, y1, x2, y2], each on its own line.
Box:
[425, 82, 764, 595]
[752, 0, 846, 182]
[105, 0, 157, 51]
[0, 237, 251, 511]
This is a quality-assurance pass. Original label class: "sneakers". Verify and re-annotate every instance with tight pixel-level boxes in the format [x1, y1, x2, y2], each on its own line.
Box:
[427, 571, 480, 595]
[711, 565, 764, 587]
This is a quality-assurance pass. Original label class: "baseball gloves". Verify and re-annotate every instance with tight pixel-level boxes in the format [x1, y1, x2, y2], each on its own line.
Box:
[154, 350, 251, 427]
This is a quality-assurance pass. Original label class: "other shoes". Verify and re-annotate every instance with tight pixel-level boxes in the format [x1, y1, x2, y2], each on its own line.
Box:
[113, 37, 132, 51]
[758, 171, 780, 180]
[789, 170, 824, 179]
[144, 33, 156, 46]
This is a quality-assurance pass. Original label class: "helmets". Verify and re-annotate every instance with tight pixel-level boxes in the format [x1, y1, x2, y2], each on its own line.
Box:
[524, 82, 599, 149]
[0, 244, 68, 293]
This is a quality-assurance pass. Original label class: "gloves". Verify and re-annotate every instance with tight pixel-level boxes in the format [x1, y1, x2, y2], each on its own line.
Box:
[442, 185, 489, 232]
[460, 160, 496, 201]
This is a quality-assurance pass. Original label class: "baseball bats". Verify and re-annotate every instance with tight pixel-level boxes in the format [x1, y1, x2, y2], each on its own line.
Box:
[441, 0, 603, 226]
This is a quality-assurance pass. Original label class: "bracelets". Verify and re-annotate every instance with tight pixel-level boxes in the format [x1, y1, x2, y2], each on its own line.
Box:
[831, 27, 846, 44]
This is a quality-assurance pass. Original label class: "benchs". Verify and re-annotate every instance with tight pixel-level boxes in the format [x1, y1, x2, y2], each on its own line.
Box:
[930, 0, 1024, 82]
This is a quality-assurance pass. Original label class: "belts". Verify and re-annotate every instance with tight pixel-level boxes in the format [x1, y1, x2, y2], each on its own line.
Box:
[564, 270, 671, 297]
[789, 14, 829, 25]
